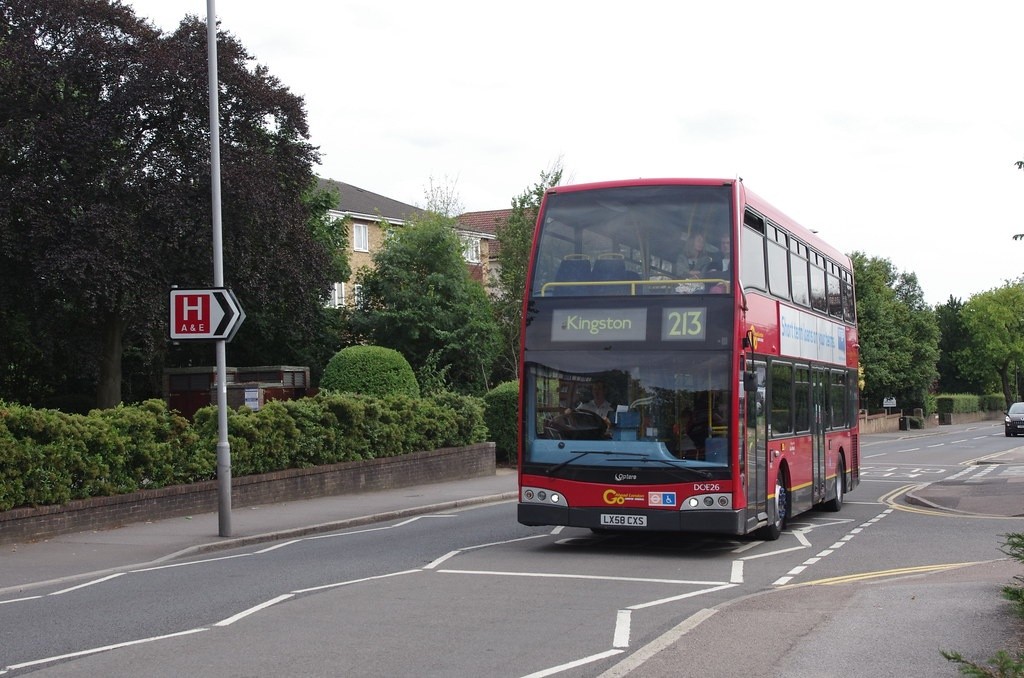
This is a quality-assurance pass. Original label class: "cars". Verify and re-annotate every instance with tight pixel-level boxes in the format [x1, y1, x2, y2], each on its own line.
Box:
[1003, 402, 1024, 437]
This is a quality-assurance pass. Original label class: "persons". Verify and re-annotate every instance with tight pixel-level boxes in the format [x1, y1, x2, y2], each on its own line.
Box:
[705, 233, 730, 273]
[675, 232, 713, 290]
[685, 380, 723, 452]
[564, 378, 616, 438]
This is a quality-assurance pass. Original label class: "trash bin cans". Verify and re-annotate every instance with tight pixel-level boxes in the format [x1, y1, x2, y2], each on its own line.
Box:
[899, 417, 910, 431]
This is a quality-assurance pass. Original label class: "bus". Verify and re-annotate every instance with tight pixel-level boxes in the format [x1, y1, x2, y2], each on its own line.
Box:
[517, 179, 862, 540]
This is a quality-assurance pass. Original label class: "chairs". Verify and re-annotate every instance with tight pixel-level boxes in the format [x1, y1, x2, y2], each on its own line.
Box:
[553, 253, 645, 296]
